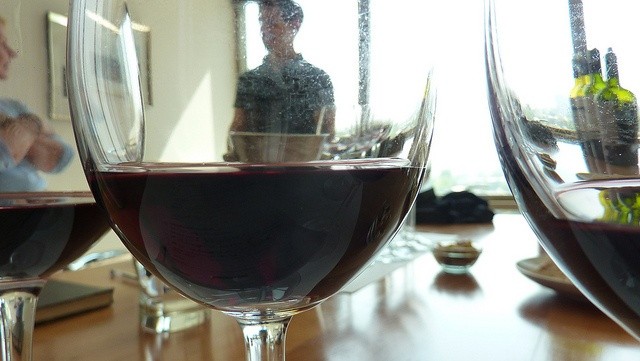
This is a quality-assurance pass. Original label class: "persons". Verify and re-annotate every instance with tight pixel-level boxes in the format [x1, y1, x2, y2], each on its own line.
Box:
[1, 13, 76, 206]
[219, 2, 337, 167]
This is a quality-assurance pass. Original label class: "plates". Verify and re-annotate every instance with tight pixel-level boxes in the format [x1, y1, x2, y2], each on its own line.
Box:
[515, 255, 579, 297]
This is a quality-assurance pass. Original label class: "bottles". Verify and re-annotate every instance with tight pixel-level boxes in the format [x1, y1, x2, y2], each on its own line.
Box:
[480, 1, 640, 343]
[569, 56, 592, 174]
[593, 47, 640, 181]
[575, 53, 598, 175]
[582, 49, 607, 174]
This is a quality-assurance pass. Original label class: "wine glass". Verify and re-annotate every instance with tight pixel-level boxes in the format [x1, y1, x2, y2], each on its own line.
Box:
[67, 0, 445, 361]
[0, 1, 144, 361]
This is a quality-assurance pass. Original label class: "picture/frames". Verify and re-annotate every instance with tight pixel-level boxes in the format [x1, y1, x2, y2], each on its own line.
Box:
[45, 9, 71, 123]
[131, 20, 157, 111]
[85, 8, 128, 100]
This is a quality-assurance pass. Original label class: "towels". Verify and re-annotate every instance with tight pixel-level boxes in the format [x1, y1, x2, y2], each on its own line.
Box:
[417, 190, 496, 223]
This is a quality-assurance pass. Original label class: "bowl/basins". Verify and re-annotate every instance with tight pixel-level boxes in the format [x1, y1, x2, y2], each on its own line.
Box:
[431, 240, 483, 275]
